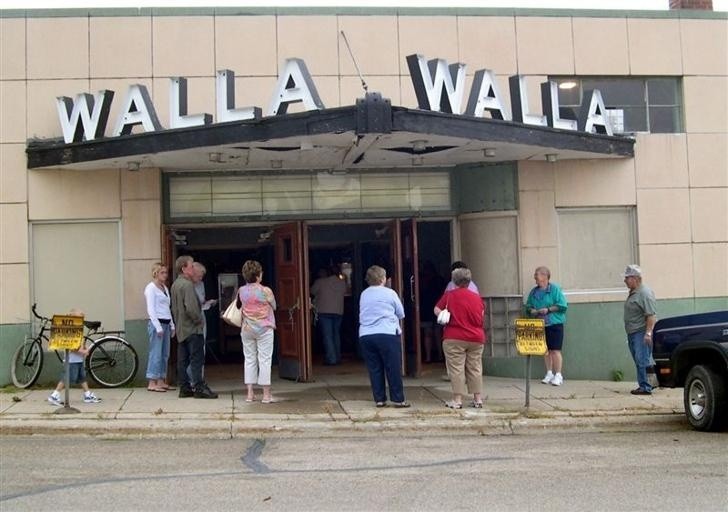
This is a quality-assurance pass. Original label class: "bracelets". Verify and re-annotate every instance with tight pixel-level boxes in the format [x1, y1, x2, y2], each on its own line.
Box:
[645, 332, 651, 337]
[547, 307, 551, 313]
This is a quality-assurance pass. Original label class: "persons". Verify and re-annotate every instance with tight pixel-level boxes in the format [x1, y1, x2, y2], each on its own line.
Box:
[310, 263, 444, 409]
[170, 254, 218, 399]
[47, 306, 102, 407]
[622, 265, 657, 395]
[143, 262, 177, 393]
[236, 260, 283, 403]
[525, 266, 568, 387]
[440, 261, 481, 383]
[434, 268, 486, 410]
[185, 261, 217, 388]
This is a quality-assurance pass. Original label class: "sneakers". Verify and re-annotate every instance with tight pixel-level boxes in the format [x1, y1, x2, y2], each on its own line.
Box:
[630, 388, 653, 395]
[468, 399, 484, 408]
[443, 400, 463, 409]
[552, 371, 564, 386]
[47, 395, 63, 407]
[178, 385, 195, 397]
[83, 392, 102, 403]
[376, 401, 411, 408]
[192, 386, 219, 399]
[541, 371, 554, 384]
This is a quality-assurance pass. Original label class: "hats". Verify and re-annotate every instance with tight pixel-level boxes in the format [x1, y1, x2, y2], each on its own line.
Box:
[620, 264, 643, 278]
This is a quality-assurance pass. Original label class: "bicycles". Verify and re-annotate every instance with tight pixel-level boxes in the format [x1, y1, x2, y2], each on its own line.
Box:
[9, 304, 138, 391]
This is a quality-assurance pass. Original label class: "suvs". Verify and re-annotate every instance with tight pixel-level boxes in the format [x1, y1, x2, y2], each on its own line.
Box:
[646, 309, 727, 429]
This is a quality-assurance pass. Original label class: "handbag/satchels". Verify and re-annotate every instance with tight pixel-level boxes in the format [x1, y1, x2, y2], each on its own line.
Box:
[222, 290, 244, 327]
[436, 291, 451, 327]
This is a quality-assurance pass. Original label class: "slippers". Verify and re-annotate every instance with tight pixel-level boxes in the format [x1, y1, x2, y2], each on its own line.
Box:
[261, 394, 279, 404]
[246, 394, 257, 403]
[161, 382, 177, 391]
[146, 386, 167, 393]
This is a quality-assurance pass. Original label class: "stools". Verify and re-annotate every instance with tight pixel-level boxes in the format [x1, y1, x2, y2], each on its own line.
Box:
[206, 338, 223, 367]
